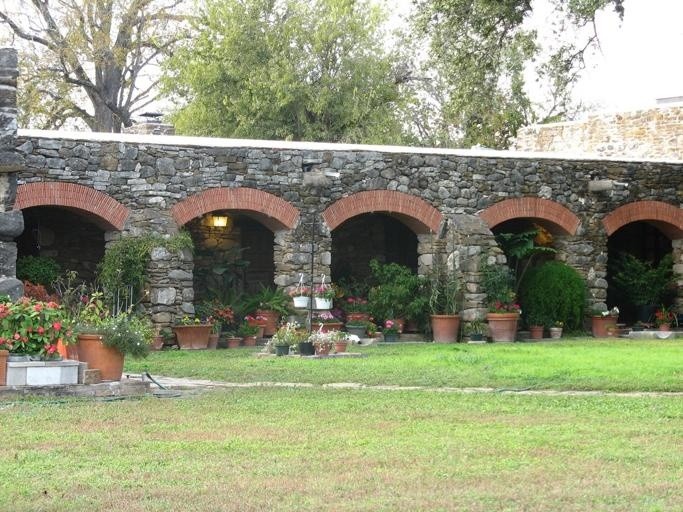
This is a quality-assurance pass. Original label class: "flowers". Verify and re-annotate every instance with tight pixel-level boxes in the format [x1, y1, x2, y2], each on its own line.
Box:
[80, 293, 150, 360]
[2, 280, 77, 361]
[308, 331, 333, 352]
[654, 302, 679, 328]
[475, 249, 522, 313]
[180, 300, 233, 332]
[588, 306, 620, 316]
[244, 315, 268, 325]
[311, 286, 335, 298]
[289, 285, 312, 297]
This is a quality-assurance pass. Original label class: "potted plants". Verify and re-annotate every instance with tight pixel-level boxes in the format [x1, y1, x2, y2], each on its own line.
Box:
[236, 322, 258, 346]
[223, 330, 243, 347]
[244, 280, 293, 336]
[427, 220, 461, 343]
[297, 330, 315, 356]
[527, 314, 564, 339]
[273, 327, 296, 356]
[332, 332, 347, 352]
[464, 316, 487, 341]
[332, 273, 403, 341]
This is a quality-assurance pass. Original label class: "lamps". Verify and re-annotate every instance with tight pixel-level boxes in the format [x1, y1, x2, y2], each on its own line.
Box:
[212, 214, 229, 226]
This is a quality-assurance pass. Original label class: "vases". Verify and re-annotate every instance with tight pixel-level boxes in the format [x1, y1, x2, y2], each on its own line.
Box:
[658, 323, 672, 331]
[591, 315, 618, 338]
[486, 313, 519, 343]
[313, 342, 334, 356]
[315, 297, 331, 309]
[292, 296, 309, 308]
[256, 327, 264, 339]
[0, 334, 125, 386]
[209, 328, 221, 348]
[173, 324, 215, 349]
